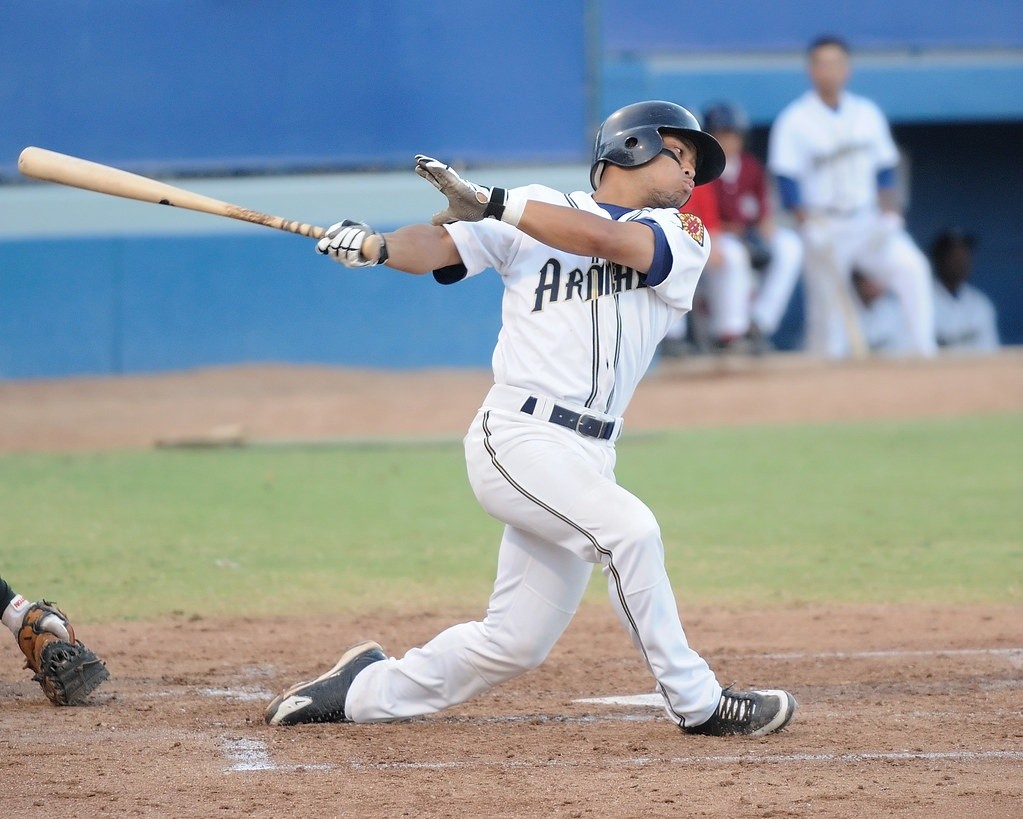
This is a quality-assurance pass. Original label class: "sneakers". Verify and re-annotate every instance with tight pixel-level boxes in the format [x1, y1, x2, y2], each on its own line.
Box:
[682, 687, 795, 739]
[16, 603, 108, 705]
[265, 639, 388, 727]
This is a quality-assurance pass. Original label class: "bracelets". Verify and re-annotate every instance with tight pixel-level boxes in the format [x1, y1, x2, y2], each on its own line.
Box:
[500, 192, 527, 228]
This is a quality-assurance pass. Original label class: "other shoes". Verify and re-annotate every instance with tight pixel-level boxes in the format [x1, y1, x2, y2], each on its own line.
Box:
[706, 334, 771, 359]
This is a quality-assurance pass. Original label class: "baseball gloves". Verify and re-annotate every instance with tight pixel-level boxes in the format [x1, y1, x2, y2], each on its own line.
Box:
[17, 600, 110, 707]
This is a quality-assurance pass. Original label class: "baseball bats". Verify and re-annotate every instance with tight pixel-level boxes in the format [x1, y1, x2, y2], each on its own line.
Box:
[18, 146, 382, 262]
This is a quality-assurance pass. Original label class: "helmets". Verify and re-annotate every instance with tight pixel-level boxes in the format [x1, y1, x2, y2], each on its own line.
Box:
[932, 226, 975, 249]
[590, 101, 726, 190]
[704, 104, 749, 135]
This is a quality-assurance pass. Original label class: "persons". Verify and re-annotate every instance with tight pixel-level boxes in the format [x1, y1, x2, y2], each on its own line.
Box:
[0, 577, 110, 706]
[264, 100, 798, 738]
[767, 36, 942, 361]
[642, 102, 999, 358]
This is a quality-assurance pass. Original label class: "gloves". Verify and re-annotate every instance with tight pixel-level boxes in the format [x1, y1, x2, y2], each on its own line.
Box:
[414, 155, 508, 225]
[315, 219, 376, 268]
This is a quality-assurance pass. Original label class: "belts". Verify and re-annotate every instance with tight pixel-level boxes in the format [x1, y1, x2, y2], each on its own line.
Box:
[521, 397, 620, 441]
